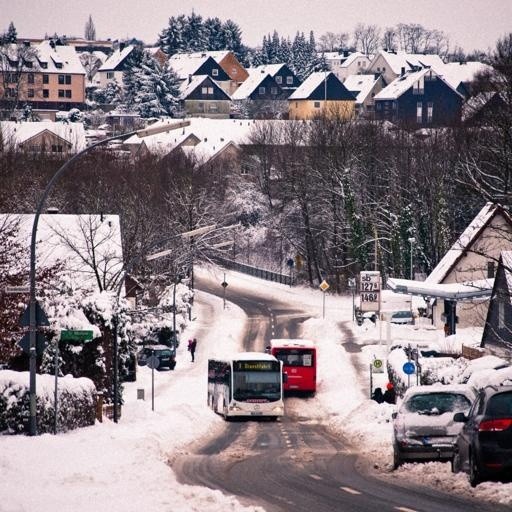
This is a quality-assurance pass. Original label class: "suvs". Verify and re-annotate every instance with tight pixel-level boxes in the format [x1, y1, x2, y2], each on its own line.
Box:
[448, 385, 512, 488]
[386, 377, 482, 468]
[133, 344, 178, 372]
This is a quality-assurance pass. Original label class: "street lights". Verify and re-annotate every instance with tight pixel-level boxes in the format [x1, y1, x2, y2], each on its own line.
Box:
[111, 222, 218, 424]
[24, 119, 190, 439]
[104, 248, 174, 295]
[407, 237, 415, 280]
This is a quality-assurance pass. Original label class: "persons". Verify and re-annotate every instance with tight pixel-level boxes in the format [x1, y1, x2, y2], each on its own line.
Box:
[372, 387, 385, 404]
[382, 383, 396, 404]
[188, 337, 197, 362]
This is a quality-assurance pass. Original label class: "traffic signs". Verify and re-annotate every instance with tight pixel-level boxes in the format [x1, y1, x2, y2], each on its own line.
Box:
[60, 329, 93, 342]
[5, 285, 31, 294]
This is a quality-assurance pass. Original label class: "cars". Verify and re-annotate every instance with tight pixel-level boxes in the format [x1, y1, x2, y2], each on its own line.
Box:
[389, 310, 415, 326]
[355, 311, 382, 330]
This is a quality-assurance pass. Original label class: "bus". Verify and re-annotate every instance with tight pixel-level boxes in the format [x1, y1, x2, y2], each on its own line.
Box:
[205, 349, 285, 425]
[265, 338, 321, 399]
